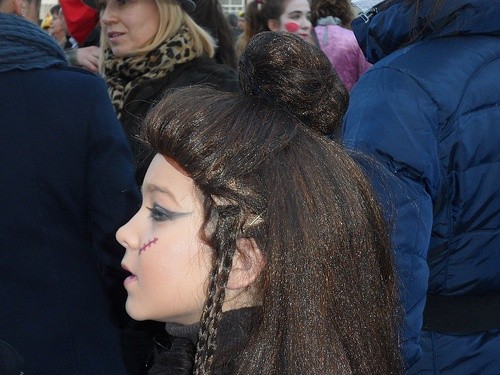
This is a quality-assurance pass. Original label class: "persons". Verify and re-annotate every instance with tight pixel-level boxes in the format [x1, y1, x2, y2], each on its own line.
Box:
[0, 0, 500, 375]
